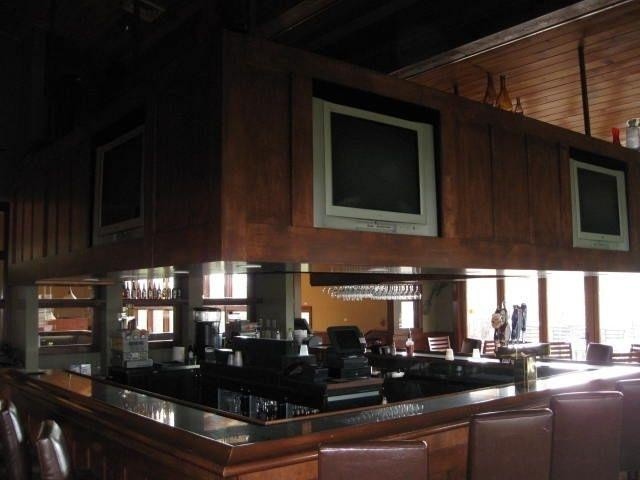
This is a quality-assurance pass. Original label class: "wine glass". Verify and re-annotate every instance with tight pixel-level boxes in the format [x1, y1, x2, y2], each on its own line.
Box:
[323, 284, 422, 301]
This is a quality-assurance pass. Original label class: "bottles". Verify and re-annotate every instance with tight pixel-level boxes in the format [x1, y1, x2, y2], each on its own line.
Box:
[405, 333, 414, 356]
[255, 327, 280, 340]
[124, 278, 172, 300]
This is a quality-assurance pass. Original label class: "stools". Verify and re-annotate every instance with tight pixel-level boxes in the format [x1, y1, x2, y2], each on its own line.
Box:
[614, 381, 639, 471]
[551, 389, 625, 477]
[319, 438, 432, 476]
[460, 408, 553, 474]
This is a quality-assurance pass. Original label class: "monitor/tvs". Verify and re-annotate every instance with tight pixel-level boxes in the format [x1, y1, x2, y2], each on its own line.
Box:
[91, 125, 145, 243]
[327, 326, 361, 353]
[568, 158, 630, 252]
[295, 319, 311, 333]
[312, 96, 440, 238]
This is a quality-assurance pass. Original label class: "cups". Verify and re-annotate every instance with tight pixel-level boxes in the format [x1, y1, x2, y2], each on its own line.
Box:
[472, 348, 481, 362]
[227, 351, 243, 367]
[445, 348, 454, 360]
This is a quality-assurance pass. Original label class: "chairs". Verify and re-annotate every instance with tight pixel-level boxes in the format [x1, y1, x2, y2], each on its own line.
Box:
[426, 333, 639, 365]
[0, 397, 96, 479]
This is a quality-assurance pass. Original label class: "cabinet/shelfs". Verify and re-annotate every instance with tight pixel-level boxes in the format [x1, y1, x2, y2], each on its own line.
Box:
[35, 294, 261, 356]
